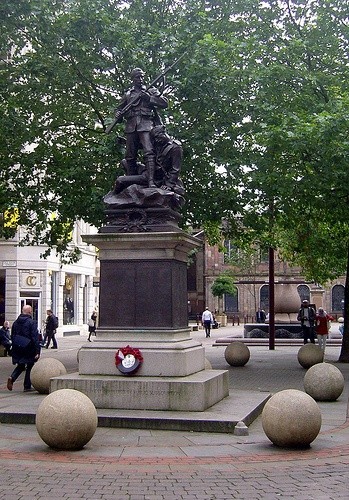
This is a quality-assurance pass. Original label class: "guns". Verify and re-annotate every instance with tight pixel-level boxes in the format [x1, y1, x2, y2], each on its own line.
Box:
[105, 52, 189, 135]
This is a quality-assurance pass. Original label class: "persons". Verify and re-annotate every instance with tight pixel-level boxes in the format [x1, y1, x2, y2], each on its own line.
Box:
[64, 297, 74, 325]
[256, 308, 266, 324]
[202, 307, 213, 338]
[114, 67, 168, 188]
[7, 305, 41, 392]
[109, 125, 182, 195]
[297, 300, 316, 345]
[87, 311, 97, 342]
[0, 321, 13, 357]
[315, 307, 334, 354]
[39, 310, 59, 349]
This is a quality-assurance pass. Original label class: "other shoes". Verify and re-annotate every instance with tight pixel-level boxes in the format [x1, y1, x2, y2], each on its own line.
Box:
[46, 345, 48, 349]
[51, 347, 57, 349]
[206, 334, 210, 338]
[87, 338, 91, 342]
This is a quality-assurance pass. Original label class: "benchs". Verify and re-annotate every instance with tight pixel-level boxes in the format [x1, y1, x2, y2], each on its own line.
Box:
[0, 344, 8, 357]
[188, 322, 221, 332]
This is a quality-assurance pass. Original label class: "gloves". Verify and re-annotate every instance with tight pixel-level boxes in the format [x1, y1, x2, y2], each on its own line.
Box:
[9, 340, 12, 344]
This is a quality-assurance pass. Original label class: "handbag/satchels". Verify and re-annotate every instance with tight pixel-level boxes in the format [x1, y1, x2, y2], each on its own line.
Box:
[14, 335, 31, 354]
[326, 320, 331, 329]
[89, 319, 95, 327]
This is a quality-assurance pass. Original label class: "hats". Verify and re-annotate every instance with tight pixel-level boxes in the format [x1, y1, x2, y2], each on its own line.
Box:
[206, 307, 208, 309]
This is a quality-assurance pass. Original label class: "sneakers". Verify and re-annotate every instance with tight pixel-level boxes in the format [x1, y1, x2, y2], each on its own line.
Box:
[24, 387, 36, 392]
[7, 377, 13, 391]
